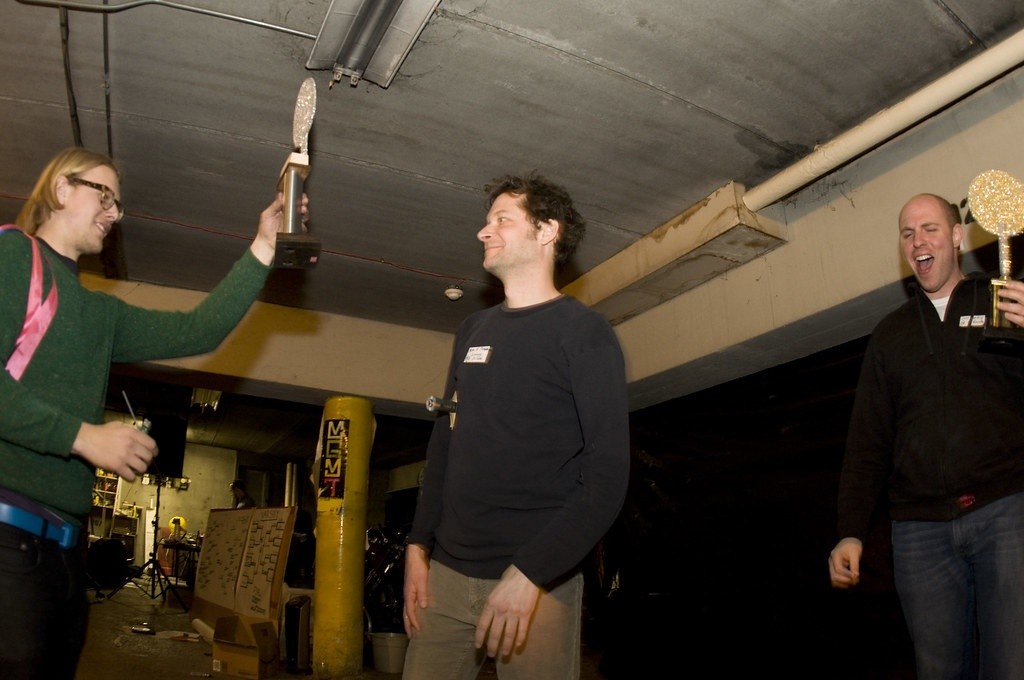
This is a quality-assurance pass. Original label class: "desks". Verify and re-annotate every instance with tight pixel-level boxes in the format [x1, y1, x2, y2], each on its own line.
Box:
[163, 544, 201, 602]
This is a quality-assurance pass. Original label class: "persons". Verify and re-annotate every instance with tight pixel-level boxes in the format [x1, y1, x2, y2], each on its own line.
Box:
[828, 191, 1024, 680]
[402, 172, 631, 680]
[0, 147, 308, 680]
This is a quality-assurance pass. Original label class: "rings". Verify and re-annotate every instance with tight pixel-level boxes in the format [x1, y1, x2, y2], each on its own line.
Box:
[404, 616, 409, 619]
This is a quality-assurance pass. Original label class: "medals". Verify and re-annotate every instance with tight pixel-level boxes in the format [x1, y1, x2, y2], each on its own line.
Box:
[968, 171, 1024, 351]
[274, 78, 322, 272]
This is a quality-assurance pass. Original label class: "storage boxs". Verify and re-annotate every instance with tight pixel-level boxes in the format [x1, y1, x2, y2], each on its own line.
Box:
[212, 616, 282, 680]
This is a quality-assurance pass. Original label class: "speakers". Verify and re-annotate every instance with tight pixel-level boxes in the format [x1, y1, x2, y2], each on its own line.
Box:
[142, 412, 188, 478]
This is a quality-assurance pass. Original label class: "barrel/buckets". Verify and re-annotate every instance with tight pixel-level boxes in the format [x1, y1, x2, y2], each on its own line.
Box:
[368, 633, 408, 673]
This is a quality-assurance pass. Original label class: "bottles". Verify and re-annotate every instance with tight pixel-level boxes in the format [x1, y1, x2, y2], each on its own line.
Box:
[131, 500, 137, 518]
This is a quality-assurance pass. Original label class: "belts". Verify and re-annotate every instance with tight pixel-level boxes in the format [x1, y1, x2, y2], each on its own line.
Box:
[0, 503, 80, 549]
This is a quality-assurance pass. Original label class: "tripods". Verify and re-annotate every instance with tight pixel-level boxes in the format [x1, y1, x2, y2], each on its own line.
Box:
[107, 477, 188, 613]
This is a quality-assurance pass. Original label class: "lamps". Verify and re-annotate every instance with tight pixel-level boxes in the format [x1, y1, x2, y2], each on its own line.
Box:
[191, 388, 222, 413]
[306, 0, 442, 88]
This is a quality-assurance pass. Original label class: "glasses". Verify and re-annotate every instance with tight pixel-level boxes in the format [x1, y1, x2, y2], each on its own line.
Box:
[72, 176, 126, 224]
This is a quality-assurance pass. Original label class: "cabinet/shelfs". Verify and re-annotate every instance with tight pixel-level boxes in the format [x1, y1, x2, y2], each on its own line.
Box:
[87, 467, 145, 568]
